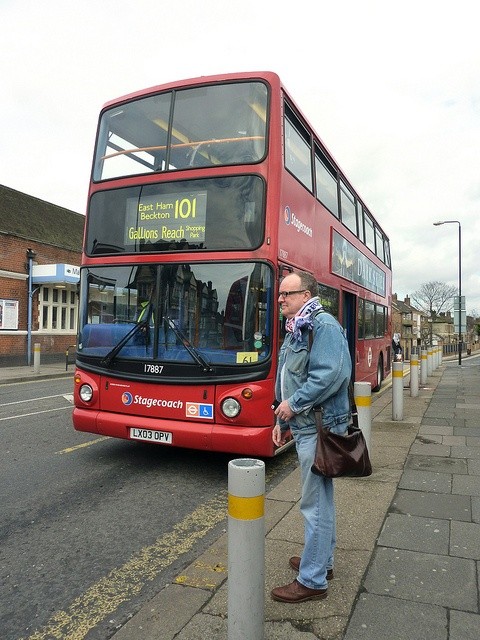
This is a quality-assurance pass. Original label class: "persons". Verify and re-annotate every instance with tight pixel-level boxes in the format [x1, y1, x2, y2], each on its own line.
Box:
[392, 332, 402, 362]
[271, 271, 372, 603]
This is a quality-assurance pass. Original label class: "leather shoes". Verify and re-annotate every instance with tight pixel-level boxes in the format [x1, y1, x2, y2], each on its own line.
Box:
[270, 579, 328, 603]
[289, 556, 334, 580]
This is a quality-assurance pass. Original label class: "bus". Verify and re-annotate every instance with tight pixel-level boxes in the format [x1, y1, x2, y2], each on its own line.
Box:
[73, 71, 392, 458]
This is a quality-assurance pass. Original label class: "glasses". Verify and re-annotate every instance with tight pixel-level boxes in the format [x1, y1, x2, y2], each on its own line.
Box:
[278, 290, 306, 297]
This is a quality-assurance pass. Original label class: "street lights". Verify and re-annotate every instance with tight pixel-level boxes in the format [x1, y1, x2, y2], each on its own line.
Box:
[433, 221, 461, 365]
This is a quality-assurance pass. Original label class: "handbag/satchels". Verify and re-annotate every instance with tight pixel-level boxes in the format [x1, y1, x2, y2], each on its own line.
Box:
[311, 425, 372, 478]
